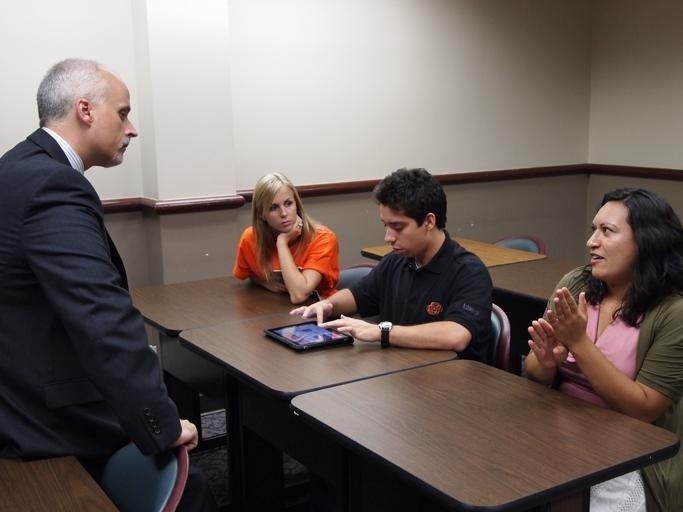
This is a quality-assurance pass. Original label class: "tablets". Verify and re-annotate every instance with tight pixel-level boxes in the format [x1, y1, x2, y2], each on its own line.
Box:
[263, 321, 353, 352]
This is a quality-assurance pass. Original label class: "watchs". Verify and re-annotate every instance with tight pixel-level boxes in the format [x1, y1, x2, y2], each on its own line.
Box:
[377, 320, 393, 348]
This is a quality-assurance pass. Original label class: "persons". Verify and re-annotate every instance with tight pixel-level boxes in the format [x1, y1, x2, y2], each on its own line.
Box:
[522, 184, 682, 511]
[230, 171, 341, 308]
[0, 57, 216, 511]
[287, 164, 493, 363]
